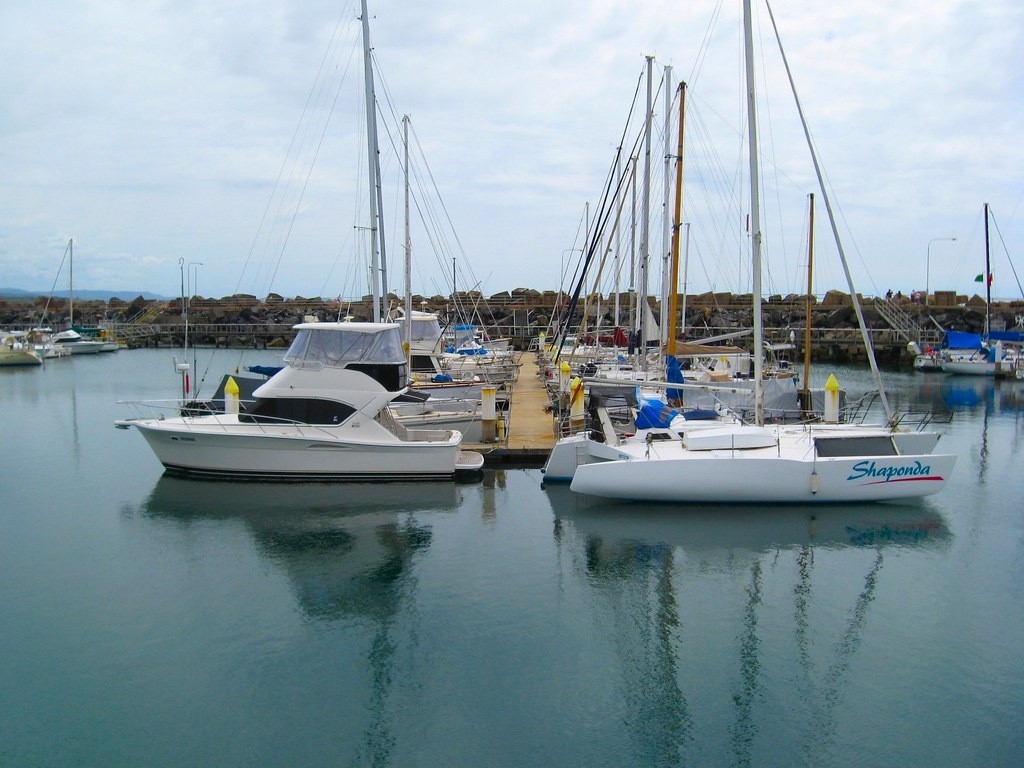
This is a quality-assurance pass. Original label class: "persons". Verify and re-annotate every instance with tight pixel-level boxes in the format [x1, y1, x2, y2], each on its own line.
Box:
[911, 290, 915, 303]
[916, 291, 921, 306]
[886, 289, 893, 302]
[897, 290, 902, 305]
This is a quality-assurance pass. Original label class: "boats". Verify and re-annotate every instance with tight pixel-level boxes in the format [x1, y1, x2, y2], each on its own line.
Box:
[1, 337, 43, 364]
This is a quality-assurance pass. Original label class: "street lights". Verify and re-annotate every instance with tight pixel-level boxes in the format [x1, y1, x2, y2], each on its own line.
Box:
[187, 262, 205, 297]
[926, 238, 957, 304]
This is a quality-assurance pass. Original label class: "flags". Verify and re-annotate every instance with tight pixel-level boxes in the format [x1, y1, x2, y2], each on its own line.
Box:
[988, 273, 993, 288]
[975, 274, 983, 282]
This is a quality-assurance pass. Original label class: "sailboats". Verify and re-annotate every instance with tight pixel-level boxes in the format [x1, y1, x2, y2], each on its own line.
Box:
[912, 201, 1024, 379]
[528, 0, 958, 503]
[114, 0, 522, 482]
[10, 238, 120, 355]
